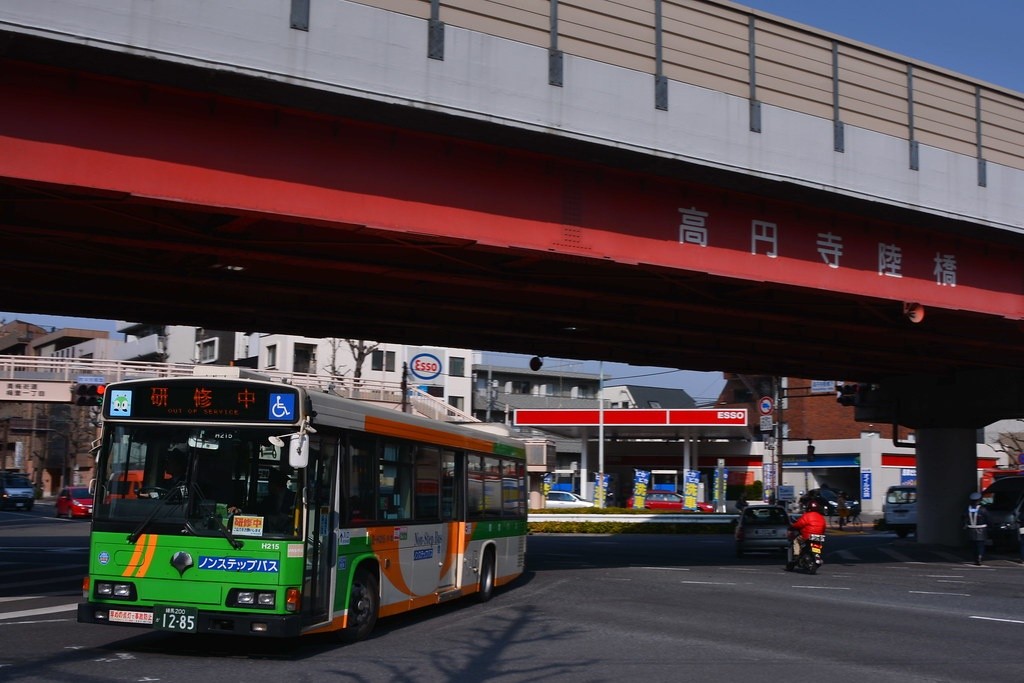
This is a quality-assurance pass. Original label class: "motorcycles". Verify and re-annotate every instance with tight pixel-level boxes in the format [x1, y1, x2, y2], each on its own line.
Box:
[786, 515, 826, 575]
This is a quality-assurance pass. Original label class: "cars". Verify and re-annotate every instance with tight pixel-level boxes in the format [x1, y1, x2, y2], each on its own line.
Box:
[626, 490, 716, 513]
[57, 486, 93, 519]
[527, 490, 595, 508]
[730, 505, 793, 563]
[798, 488, 861, 517]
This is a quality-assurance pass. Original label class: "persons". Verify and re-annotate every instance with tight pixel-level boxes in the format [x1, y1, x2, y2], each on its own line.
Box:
[790, 501, 826, 565]
[965, 493, 994, 564]
[227, 468, 298, 526]
[837, 491, 850, 526]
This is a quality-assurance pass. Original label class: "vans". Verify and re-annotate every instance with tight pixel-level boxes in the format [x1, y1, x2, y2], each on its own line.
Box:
[105, 471, 173, 503]
[0, 474, 36, 512]
[885, 485, 918, 539]
[976, 475, 1024, 553]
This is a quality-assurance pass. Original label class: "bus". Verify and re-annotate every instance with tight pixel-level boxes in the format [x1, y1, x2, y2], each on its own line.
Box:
[77, 366, 527, 642]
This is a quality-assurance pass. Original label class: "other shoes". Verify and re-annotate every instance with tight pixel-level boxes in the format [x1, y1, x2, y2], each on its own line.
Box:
[792, 556, 798, 561]
[847, 521, 851, 522]
[840, 528, 842, 530]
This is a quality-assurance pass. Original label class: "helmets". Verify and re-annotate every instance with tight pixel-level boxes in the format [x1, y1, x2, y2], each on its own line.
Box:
[808, 500, 819, 510]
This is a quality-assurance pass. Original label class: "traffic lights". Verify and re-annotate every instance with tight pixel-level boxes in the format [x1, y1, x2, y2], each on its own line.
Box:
[75, 383, 106, 405]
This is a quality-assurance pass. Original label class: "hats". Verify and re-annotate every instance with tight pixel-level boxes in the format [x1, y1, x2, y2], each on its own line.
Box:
[969, 492, 982, 500]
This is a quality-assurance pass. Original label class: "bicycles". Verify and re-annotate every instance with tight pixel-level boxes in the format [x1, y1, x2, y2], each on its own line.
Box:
[830, 509, 862, 532]
